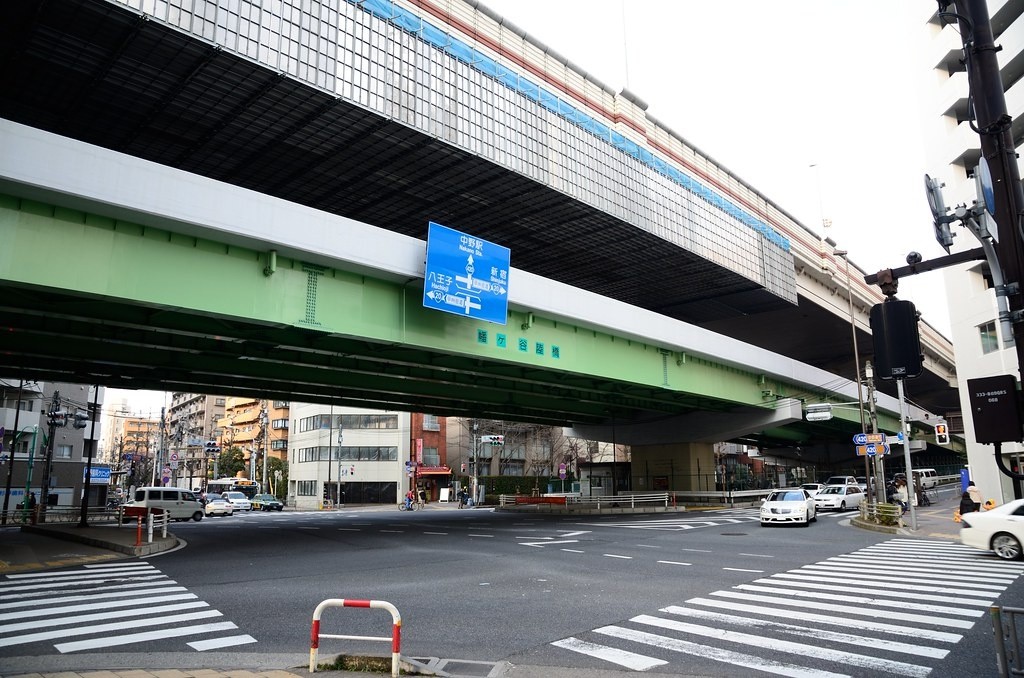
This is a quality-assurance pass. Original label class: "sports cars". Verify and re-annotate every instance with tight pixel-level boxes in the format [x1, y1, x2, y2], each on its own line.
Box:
[205, 498, 235, 517]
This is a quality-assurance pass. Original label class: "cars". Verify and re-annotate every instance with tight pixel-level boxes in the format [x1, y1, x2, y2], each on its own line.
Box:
[191, 491, 251, 511]
[959, 498, 1024, 560]
[109, 487, 128, 503]
[251, 494, 284, 511]
[799, 475, 877, 513]
[114, 499, 135, 524]
[760, 489, 817, 527]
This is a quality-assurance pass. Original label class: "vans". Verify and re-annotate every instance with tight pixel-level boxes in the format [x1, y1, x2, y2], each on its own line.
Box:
[134, 487, 204, 522]
[903, 469, 939, 489]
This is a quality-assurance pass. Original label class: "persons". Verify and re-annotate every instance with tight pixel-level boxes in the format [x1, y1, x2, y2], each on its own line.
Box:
[414, 488, 426, 511]
[966, 480, 982, 512]
[960, 491, 974, 528]
[897, 480, 908, 515]
[457, 489, 464, 509]
[888, 482, 896, 504]
[409, 491, 415, 508]
[405, 488, 414, 510]
[21, 492, 36, 522]
[982, 498, 996, 511]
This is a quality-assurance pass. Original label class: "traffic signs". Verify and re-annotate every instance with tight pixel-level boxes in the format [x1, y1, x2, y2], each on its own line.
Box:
[424, 221, 510, 326]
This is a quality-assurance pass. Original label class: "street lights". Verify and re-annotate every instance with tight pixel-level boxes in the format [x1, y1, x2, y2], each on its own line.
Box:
[9, 423, 49, 523]
[832, 250, 874, 521]
[225, 425, 255, 483]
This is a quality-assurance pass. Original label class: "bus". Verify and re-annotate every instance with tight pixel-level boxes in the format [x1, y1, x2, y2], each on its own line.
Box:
[207, 478, 261, 500]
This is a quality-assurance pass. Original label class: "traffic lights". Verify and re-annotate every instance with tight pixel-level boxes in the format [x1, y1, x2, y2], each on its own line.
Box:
[461, 463, 465, 473]
[489, 442, 502, 446]
[204, 442, 218, 447]
[47, 411, 66, 428]
[490, 437, 502, 441]
[73, 411, 89, 430]
[206, 447, 220, 452]
[935, 424, 950, 445]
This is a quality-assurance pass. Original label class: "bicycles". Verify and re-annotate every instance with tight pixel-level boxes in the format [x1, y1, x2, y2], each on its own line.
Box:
[12, 501, 39, 523]
[398, 498, 419, 511]
[887, 489, 910, 516]
[917, 491, 930, 507]
